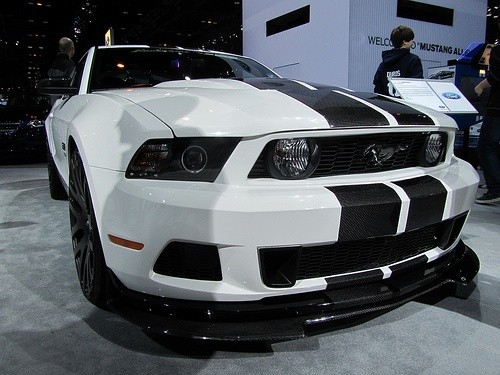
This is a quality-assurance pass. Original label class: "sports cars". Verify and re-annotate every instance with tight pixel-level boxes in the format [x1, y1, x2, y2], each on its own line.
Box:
[45, 44, 481, 344]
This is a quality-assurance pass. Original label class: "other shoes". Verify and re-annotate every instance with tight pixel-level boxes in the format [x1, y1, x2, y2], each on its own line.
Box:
[475, 191, 500, 203]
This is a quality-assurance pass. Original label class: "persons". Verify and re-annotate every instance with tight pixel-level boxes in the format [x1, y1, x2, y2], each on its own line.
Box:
[48, 37, 78, 109]
[373, 25, 424, 99]
[474, 44, 500, 203]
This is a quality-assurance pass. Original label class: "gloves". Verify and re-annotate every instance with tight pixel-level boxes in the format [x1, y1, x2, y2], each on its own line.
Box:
[474, 79, 491, 96]
